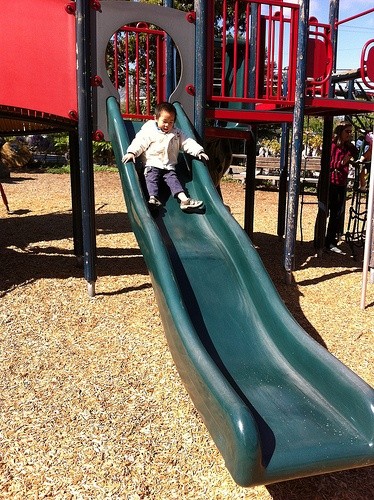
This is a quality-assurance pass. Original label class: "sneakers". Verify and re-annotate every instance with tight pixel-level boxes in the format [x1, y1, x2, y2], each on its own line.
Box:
[179, 199, 203, 210]
[316, 245, 343, 257]
[147, 196, 163, 210]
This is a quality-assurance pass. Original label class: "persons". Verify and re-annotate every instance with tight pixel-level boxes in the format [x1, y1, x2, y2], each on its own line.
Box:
[313, 120, 374, 254]
[121, 102, 210, 210]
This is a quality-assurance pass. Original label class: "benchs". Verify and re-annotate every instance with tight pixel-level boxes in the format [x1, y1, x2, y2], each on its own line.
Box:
[256, 157, 321, 182]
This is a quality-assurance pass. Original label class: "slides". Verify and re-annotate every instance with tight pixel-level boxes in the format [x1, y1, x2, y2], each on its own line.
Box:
[106, 97, 374, 488]
